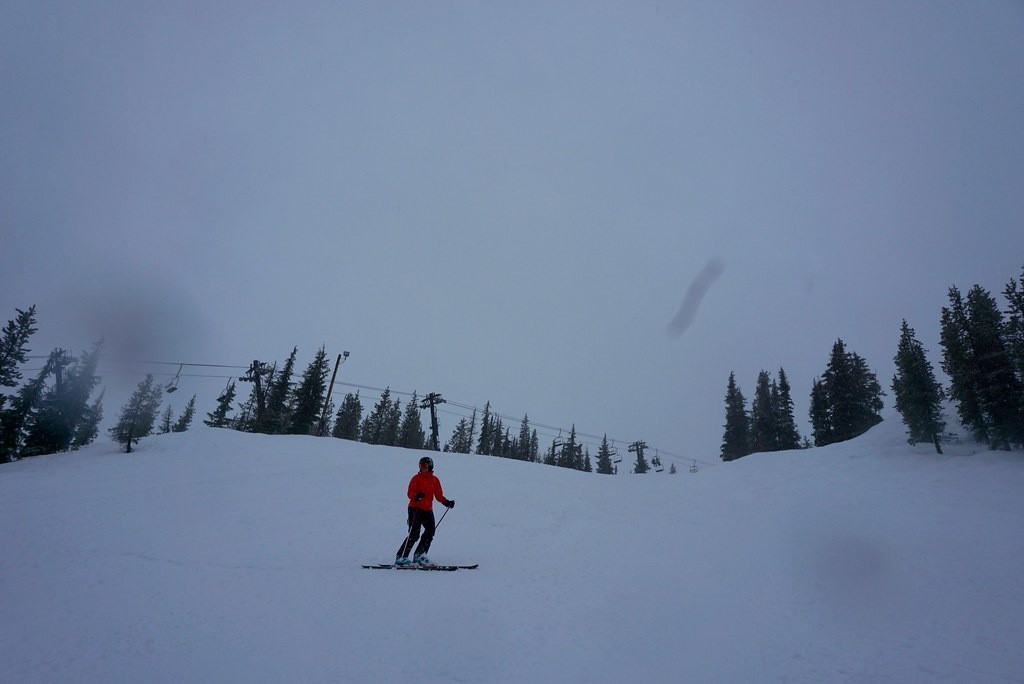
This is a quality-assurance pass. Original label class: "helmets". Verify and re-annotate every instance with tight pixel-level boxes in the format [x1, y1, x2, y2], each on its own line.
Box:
[418, 456, 434, 471]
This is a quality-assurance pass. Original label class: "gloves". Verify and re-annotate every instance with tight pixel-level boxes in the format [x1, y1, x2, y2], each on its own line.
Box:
[445, 500, 454, 508]
[414, 493, 424, 500]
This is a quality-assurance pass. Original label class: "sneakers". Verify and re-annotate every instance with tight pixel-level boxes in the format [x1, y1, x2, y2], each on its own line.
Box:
[394, 555, 419, 567]
[412, 555, 434, 567]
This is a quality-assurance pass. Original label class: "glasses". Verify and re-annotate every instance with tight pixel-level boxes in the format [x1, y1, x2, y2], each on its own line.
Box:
[419, 464, 427, 468]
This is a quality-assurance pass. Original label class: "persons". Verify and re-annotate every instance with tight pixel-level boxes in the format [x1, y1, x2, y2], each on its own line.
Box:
[395, 456, 454, 566]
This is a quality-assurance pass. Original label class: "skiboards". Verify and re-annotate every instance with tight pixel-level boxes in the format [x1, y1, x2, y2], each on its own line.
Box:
[360, 563, 480, 572]
